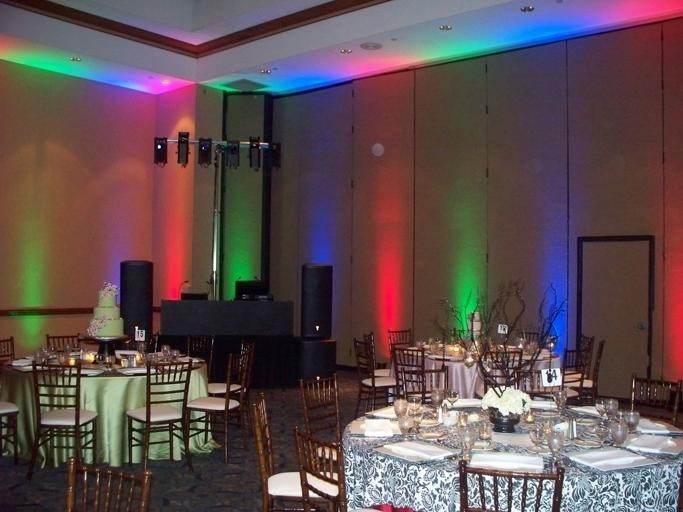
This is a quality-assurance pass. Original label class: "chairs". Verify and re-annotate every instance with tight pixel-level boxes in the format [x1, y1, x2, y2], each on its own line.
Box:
[186, 354, 251, 463]
[30, 358, 100, 473]
[630, 374, 681, 427]
[234, 282, 271, 302]
[342, 404, 683, 510]
[125, 359, 193, 472]
[1, 400, 21, 471]
[1, 331, 217, 393]
[181, 291, 208, 301]
[352, 329, 605, 418]
[207, 337, 255, 437]
[66, 457, 151, 511]
[299, 372, 345, 468]
[252, 392, 347, 511]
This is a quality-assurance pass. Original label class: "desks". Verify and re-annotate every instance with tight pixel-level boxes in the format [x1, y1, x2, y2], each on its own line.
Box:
[159, 301, 294, 342]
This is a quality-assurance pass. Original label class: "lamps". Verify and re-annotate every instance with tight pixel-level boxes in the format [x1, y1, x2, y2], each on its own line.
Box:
[152, 131, 281, 170]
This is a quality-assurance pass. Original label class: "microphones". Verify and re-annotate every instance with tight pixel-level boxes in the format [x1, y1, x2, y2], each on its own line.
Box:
[177, 281, 189, 300]
[254, 276, 266, 301]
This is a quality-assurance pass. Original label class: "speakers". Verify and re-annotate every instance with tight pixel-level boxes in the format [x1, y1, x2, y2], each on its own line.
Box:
[121, 261, 153, 335]
[302, 264, 333, 340]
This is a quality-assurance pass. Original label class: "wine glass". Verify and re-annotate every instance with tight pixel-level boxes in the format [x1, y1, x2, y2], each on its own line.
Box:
[394, 389, 640, 461]
[414, 336, 557, 358]
[33, 343, 179, 370]
